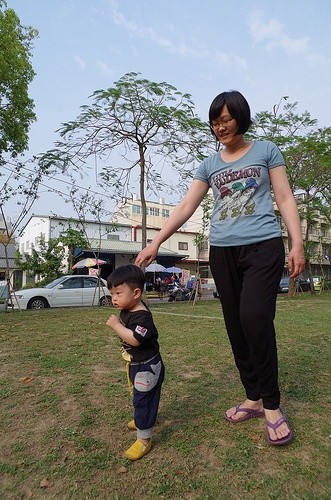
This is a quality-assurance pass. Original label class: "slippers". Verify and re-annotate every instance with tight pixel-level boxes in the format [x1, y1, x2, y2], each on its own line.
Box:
[265, 407, 292, 444]
[224, 400, 265, 424]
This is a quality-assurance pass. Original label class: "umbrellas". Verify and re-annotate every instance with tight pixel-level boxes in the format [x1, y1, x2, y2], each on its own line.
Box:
[162, 266, 183, 276]
[71, 258, 107, 269]
[144, 263, 166, 283]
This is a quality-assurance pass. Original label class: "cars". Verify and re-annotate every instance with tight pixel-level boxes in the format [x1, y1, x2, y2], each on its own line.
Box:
[278, 275, 311, 293]
[6, 274, 112, 310]
[307, 275, 331, 291]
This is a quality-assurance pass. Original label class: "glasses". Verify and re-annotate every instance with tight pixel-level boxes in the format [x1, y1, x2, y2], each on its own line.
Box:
[209, 118, 236, 130]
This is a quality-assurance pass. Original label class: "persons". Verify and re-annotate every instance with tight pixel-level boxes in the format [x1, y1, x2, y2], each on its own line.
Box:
[155, 274, 181, 295]
[105, 265, 164, 461]
[134, 90, 306, 445]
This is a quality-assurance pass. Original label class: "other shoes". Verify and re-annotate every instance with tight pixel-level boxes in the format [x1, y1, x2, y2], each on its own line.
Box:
[127, 420, 137, 430]
[124, 437, 152, 460]
